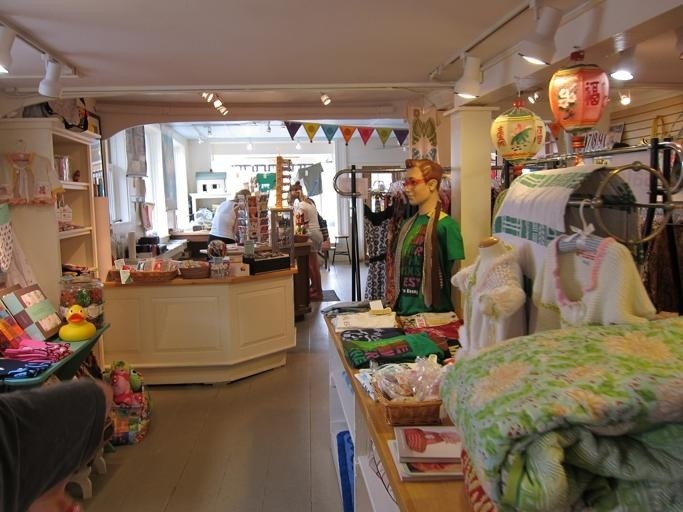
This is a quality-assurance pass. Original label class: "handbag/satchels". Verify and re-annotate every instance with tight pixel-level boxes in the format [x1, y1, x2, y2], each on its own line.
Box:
[103, 368, 153, 446]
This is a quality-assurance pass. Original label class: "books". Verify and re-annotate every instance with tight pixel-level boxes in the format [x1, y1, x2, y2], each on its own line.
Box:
[394, 426, 465, 463]
[387, 440, 465, 482]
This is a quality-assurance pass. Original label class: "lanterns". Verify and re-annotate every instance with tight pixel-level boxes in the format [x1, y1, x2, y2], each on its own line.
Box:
[549, 51, 609, 154]
[490, 99, 546, 176]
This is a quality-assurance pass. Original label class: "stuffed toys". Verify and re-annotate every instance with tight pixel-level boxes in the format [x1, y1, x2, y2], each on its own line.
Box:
[102, 359, 144, 404]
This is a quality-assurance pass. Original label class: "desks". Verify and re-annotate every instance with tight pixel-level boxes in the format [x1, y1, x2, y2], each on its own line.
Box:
[0, 322, 110, 386]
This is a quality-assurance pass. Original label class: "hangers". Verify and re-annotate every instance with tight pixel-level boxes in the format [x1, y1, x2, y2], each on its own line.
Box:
[556, 199, 603, 254]
[439, 178, 449, 190]
[391, 179, 407, 202]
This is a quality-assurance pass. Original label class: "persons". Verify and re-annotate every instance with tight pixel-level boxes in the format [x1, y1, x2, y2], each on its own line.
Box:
[287, 181, 323, 299]
[208, 190, 251, 245]
[393, 159, 465, 315]
[0, 377, 113, 512]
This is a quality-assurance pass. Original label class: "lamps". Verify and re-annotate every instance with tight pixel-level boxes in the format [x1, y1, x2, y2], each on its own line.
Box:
[517, 0, 563, 65]
[620, 92, 631, 105]
[609, 34, 637, 81]
[126, 160, 149, 177]
[321, 95, 331, 106]
[202, 93, 228, 115]
[528, 93, 539, 104]
[452, 52, 483, 99]
[0, 19, 78, 97]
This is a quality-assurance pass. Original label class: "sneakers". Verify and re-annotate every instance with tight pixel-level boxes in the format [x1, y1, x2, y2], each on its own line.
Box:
[310, 285, 323, 301]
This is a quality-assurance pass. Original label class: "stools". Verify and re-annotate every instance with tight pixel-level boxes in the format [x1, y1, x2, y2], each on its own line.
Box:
[332, 235, 351, 264]
[317, 250, 330, 270]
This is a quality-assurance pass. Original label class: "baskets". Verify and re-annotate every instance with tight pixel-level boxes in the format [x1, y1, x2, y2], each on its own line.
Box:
[374, 383, 442, 427]
[130, 270, 178, 283]
[109, 271, 121, 282]
[180, 268, 210, 279]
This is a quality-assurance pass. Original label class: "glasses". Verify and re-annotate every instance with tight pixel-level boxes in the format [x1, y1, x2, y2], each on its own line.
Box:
[402, 178, 425, 186]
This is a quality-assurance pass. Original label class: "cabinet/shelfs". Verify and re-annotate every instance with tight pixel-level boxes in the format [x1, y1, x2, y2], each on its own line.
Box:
[324, 313, 474, 512]
[0, 117, 105, 373]
